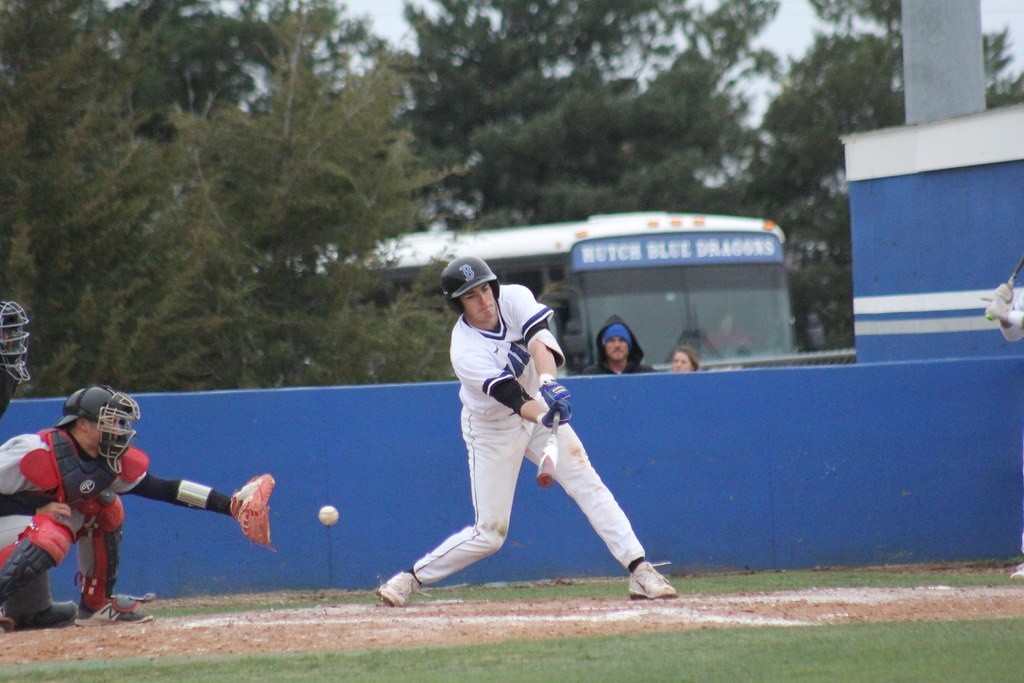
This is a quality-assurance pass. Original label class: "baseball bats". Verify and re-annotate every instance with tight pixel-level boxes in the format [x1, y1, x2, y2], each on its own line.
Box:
[537, 409, 561, 489]
[985, 256, 1023, 322]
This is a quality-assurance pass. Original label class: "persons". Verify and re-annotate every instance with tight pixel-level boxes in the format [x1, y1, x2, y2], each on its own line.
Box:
[0, 385, 275, 632]
[672, 346, 699, 371]
[581, 313, 657, 373]
[376, 256, 677, 607]
[705, 312, 752, 359]
[981, 283, 1024, 342]
[0, 301, 31, 414]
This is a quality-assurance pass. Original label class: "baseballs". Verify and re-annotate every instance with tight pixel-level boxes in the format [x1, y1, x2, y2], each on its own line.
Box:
[318, 506, 339, 527]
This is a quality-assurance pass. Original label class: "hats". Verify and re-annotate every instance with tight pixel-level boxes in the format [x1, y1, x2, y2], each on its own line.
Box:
[601, 323, 631, 350]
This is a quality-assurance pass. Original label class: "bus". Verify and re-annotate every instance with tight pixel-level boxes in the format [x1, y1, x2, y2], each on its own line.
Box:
[315, 209, 800, 374]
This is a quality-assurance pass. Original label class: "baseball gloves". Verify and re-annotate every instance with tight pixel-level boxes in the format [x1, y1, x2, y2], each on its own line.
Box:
[230, 475, 274, 544]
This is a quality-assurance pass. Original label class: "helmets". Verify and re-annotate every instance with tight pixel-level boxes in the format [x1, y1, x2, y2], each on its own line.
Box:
[0, 301, 28, 367]
[52, 386, 133, 427]
[440, 256, 499, 314]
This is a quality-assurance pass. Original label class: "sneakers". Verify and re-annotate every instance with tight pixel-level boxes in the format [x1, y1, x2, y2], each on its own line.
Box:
[0, 600, 76, 633]
[376, 571, 421, 608]
[629, 561, 678, 599]
[74, 591, 154, 625]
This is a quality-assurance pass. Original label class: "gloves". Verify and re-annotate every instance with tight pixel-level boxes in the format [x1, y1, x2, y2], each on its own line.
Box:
[985, 283, 1016, 324]
[538, 378, 573, 427]
